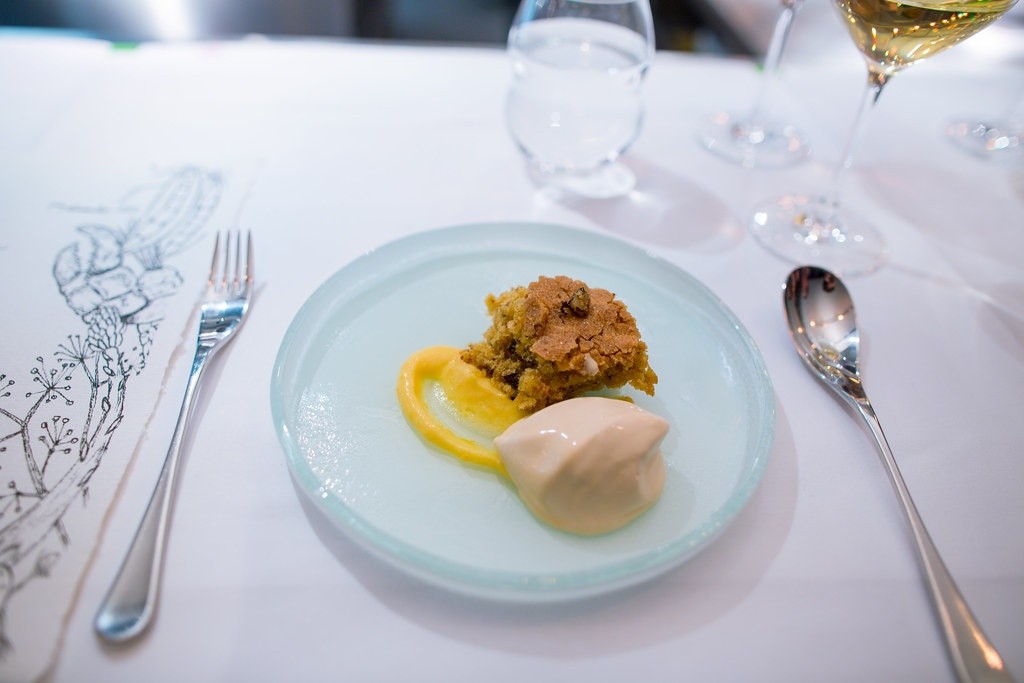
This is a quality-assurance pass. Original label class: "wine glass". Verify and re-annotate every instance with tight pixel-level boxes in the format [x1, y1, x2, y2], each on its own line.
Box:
[751, 1, 1011, 281]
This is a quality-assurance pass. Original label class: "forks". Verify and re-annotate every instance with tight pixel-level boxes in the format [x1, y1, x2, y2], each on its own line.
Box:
[94, 228, 259, 642]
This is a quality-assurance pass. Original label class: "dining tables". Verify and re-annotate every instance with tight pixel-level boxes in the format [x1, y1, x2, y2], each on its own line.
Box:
[1, 30, 1021, 683]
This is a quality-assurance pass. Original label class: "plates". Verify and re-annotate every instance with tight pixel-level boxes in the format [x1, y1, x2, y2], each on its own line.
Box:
[271, 220, 776, 604]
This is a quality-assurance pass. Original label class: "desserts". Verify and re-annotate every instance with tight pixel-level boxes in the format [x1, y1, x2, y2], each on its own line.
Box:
[394, 270, 663, 538]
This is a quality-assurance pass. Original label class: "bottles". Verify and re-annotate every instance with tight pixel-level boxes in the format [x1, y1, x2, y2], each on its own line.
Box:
[504, 1, 658, 170]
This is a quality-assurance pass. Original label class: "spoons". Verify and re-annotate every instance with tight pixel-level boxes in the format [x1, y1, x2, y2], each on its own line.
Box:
[783, 263, 1011, 683]
